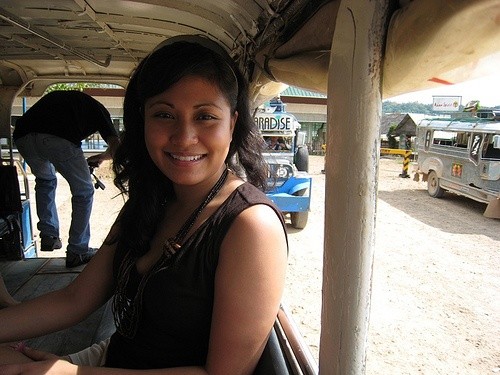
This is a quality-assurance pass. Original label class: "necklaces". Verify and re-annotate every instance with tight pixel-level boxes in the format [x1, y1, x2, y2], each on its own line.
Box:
[111, 169, 229, 339]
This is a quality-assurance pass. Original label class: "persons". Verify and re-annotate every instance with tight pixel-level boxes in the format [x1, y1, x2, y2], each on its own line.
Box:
[387, 134, 396, 148]
[0, 35, 289, 375]
[405, 136, 411, 149]
[0, 272, 31, 354]
[13, 90, 121, 268]
[264, 131, 287, 150]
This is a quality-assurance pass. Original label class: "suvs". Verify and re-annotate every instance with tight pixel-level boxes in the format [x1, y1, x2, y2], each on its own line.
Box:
[230, 100, 312, 230]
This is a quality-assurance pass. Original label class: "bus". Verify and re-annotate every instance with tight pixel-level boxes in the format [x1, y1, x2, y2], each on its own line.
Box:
[413, 118, 500, 219]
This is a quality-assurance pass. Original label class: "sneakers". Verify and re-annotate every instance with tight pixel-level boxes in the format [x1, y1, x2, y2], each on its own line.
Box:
[66, 247, 100, 268]
[40, 236, 62, 251]
